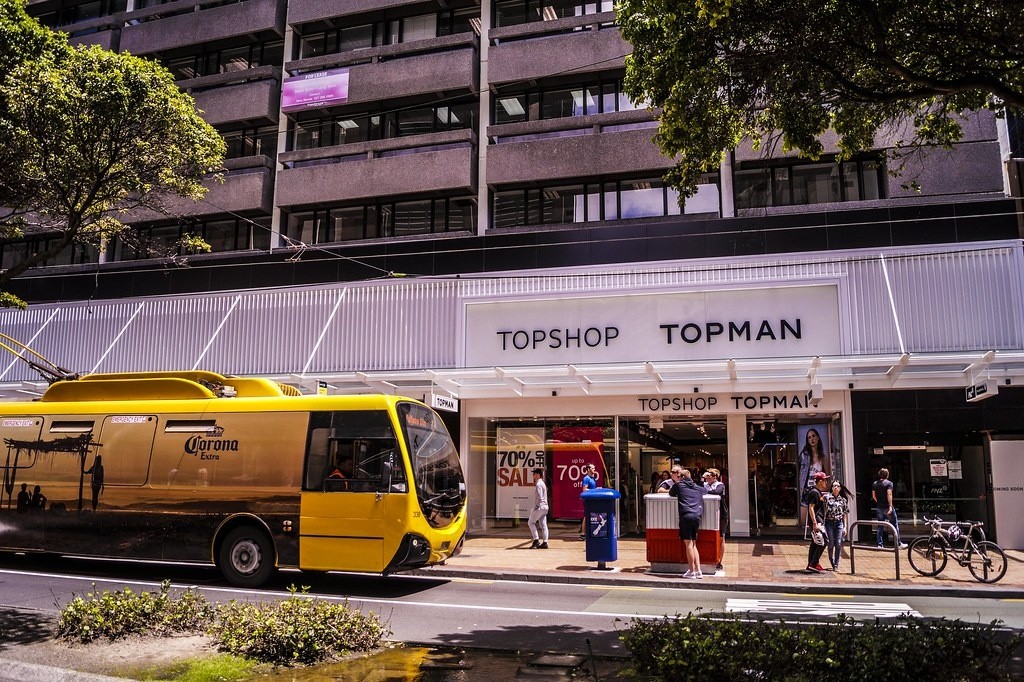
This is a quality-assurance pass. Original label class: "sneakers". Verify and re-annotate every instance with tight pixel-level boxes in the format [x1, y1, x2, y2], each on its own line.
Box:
[898, 543, 908, 550]
[808, 563, 825, 572]
[696, 571, 703, 579]
[537, 544, 548, 549]
[531, 541, 540, 548]
[683, 570, 696, 578]
[805, 566, 816, 572]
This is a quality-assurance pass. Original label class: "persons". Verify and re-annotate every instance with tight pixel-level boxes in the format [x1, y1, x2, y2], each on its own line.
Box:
[798, 428, 830, 529]
[16, 483, 47, 514]
[824, 480, 855, 571]
[805, 471, 829, 574]
[327, 453, 374, 492]
[872, 468, 908, 550]
[649, 464, 682, 494]
[701, 468, 728, 570]
[528, 467, 550, 550]
[579, 464, 597, 541]
[83, 455, 104, 512]
[669, 469, 708, 579]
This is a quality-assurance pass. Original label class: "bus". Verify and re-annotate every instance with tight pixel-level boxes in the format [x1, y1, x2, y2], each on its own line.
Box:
[0, 333, 468, 592]
[470, 440, 629, 525]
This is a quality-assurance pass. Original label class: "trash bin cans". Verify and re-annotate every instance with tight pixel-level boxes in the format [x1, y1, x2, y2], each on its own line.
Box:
[579, 488, 621, 562]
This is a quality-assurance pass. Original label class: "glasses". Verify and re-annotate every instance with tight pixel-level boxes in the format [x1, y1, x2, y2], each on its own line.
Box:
[671, 471, 677, 474]
[677, 475, 682, 478]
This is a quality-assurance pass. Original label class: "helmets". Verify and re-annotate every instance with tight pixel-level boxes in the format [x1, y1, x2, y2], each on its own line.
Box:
[947, 525, 961, 541]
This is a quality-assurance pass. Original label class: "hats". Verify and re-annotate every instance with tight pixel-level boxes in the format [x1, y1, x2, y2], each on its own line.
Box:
[531, 468, 542, 474]
[814, 472, 830, 479]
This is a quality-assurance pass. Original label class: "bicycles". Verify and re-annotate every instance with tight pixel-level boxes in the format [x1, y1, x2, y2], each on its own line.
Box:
[907, 516, 1008, 583]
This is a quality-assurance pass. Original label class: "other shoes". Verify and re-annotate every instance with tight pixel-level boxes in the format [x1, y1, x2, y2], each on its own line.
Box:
[579, 536, 586, 541]
